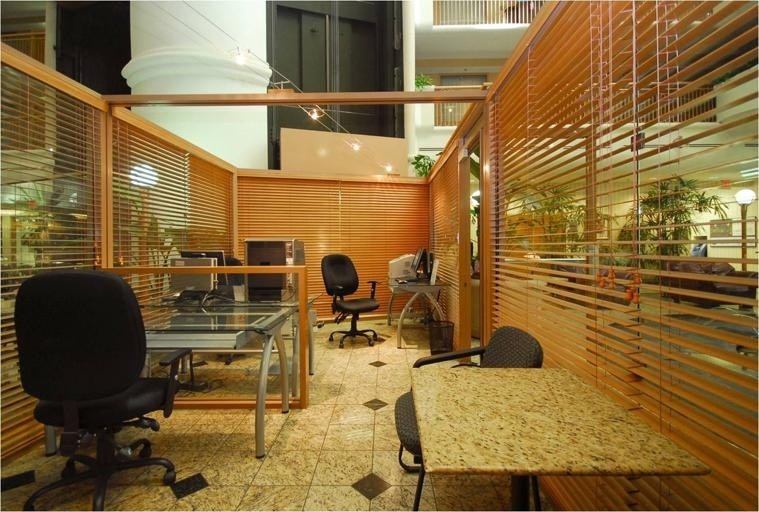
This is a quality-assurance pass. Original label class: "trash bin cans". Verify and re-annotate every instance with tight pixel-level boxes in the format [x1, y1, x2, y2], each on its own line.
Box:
[429, 321, 454, 355]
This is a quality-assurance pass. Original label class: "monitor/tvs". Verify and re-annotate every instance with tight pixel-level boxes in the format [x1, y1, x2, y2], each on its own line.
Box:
[411, 247, 424, 280]
[180, 249, 228, 286]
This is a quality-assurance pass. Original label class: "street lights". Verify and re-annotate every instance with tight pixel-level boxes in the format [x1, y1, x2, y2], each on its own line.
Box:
[127, 162, 162, 310]
[732, 187, 756, 275]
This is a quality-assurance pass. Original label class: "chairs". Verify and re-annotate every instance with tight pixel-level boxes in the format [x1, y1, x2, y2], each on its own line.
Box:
[321, 253, 380, 349]
[14, 267, 191, 511]
[550, 256, 759, 316]
[393, 324, 542, 511]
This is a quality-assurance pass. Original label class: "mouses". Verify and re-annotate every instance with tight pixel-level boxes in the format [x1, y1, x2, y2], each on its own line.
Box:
[399, 280, 407, 284]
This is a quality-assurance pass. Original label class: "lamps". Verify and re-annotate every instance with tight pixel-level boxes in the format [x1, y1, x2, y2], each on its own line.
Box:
[735, 189, 756, 275]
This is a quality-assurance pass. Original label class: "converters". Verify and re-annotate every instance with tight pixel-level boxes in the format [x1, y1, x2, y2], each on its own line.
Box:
[184, 379, 208, 392]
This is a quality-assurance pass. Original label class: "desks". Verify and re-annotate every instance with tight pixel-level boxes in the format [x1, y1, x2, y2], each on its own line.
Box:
[123, 288, 323, 460]
[387, 273, 450, 348]
[410, 366, 711, 511]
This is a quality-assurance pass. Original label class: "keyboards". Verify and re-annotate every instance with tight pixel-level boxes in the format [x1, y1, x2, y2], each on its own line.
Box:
[395, 275, 416, 282]
